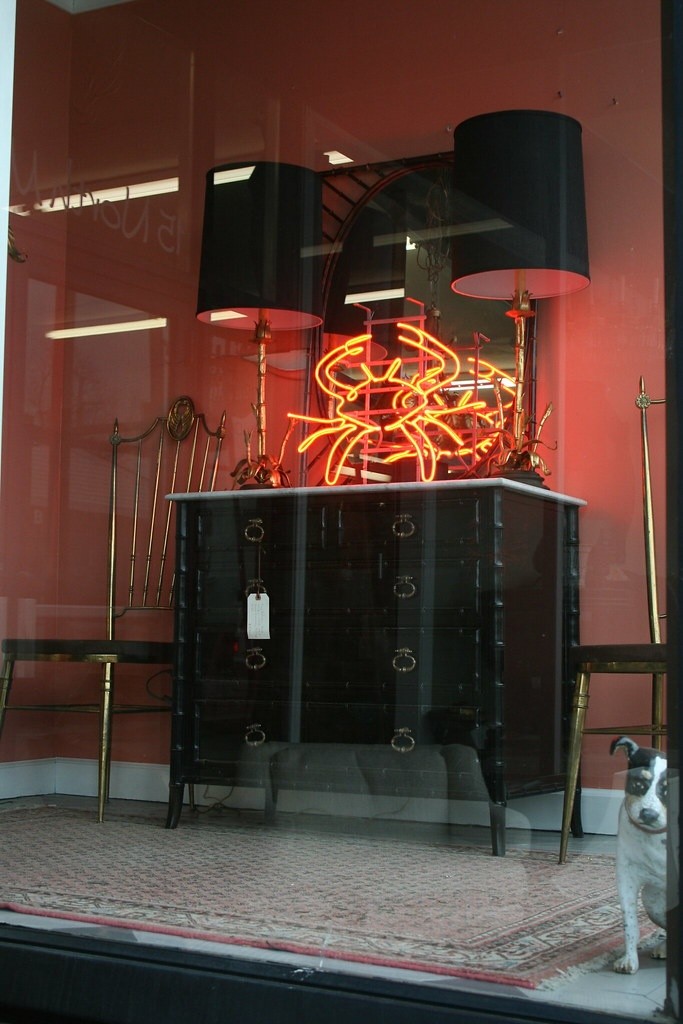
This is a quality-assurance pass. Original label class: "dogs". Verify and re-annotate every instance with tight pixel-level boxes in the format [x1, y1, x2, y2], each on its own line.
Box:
[610, 734, 669, 973]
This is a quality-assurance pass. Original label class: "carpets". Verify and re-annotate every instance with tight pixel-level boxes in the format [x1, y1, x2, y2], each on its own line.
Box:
[0, 807, 667, 991]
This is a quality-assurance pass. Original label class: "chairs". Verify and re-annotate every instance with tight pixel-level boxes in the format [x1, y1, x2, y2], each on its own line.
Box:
[0, 395, 227, 821]
[556, 376, 670, 864]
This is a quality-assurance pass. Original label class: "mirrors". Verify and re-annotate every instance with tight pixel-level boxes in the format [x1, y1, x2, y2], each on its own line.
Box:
[300, 151, 535, 487]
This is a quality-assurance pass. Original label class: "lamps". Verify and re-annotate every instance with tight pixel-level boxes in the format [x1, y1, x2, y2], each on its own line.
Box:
[450, 109, 589, 482]
[195, 160, 324, 490]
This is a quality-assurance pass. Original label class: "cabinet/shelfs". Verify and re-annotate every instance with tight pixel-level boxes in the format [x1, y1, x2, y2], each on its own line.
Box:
[165, 478, 588, 855]
